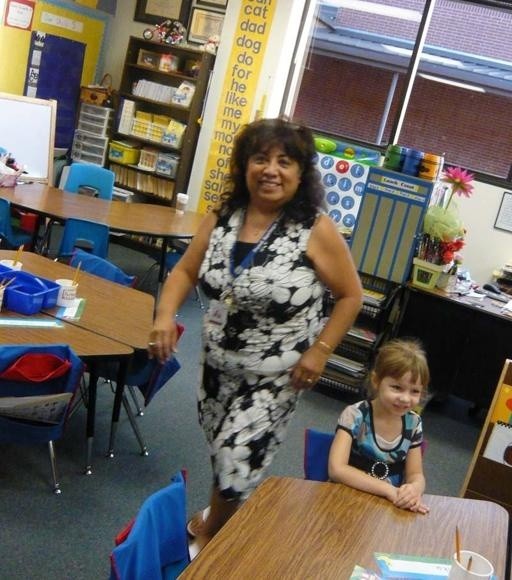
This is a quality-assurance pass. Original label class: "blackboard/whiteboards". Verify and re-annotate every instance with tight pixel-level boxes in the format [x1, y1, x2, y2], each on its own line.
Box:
[0, 92, 57, 184]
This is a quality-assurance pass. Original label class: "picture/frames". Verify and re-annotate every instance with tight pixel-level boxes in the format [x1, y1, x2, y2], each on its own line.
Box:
[186, 7, 225, 48]
[196, 0, 229, 9]
[133, 0, 193, 32]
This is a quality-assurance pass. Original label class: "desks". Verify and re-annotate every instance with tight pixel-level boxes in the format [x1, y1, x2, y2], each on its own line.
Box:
[0, 182, 207, 280]
[174, 476, 509, 580]
[389, 272, 511, 422]
[0, 249, 156, 476]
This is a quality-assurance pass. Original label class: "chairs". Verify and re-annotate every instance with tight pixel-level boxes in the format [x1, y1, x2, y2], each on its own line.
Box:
[58, 162, 116, 260]
[67, 249, 137, 289]
[102, 321, 184, 460]
[303, 428, 432, 485]
[107, 466, 191, 579]
[0, 342, 86, 495]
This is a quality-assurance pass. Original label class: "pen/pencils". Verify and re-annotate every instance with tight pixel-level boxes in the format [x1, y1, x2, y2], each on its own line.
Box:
[0, 277, 16, 289]
[72, 260, 82, 286]
[456, 526, 460, 563]
[13, 245, 24, 267]
[467, 556, 472, 570]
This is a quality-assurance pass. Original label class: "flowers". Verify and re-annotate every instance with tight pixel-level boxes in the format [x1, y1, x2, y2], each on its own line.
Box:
[418, 166, 477, 266]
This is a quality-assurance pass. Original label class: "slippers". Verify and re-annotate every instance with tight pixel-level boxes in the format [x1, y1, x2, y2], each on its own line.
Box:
[186, 505, 211, 561]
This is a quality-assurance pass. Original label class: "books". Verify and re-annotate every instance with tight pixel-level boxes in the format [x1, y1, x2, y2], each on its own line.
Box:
[320, 287, 386, 388]
[107, 78, 196, 200]
[501, 300, 512, 317]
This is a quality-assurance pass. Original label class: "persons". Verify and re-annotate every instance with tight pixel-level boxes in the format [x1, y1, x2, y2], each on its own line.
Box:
[327, 338, 430, 515]
[147, 116, 363, 561]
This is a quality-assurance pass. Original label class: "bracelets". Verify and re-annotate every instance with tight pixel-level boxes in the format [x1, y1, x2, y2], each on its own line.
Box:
[315, 339, 335, 352]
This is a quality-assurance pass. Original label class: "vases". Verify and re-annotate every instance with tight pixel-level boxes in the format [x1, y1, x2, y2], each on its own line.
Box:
[413, 259, 465, 294]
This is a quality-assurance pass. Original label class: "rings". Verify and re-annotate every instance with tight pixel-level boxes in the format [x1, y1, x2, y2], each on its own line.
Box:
[148, 343, 156, 347]
[307, 379, 312, 384]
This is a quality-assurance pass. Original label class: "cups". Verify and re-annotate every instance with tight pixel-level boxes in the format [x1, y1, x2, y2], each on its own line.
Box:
[16, 211, 39, 253]
[159, 54, 180, 73]
[381, 144, 444, 180]
[175, 192, 189, 214]
[2, 259, 23, 271]
[449, 550, 495, 579]
[55, 278, 78, 307]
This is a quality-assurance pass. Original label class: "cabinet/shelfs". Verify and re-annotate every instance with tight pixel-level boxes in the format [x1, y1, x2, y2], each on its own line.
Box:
[315, 269, 409, 397]
[104, 34, 217, 207]
